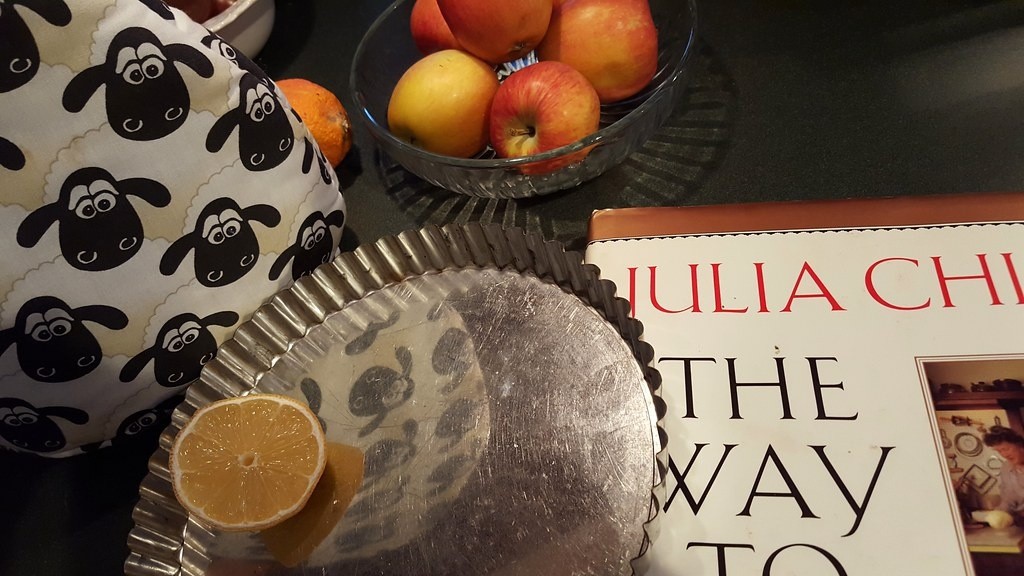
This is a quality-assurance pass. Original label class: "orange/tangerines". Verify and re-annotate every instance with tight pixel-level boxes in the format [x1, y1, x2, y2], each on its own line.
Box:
[272, 78, 353, 168]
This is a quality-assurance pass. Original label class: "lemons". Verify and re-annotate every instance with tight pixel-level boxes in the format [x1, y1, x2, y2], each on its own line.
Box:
[259, 443, 360, 567]
[169, 394, 327, 531]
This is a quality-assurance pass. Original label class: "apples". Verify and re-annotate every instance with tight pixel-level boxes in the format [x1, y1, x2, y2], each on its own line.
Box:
[387, 0, 659, 177]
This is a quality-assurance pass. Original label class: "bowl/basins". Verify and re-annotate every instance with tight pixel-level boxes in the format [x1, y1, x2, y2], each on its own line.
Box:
[201, 0, 275, 60]
[349, 0, 697, 198]
